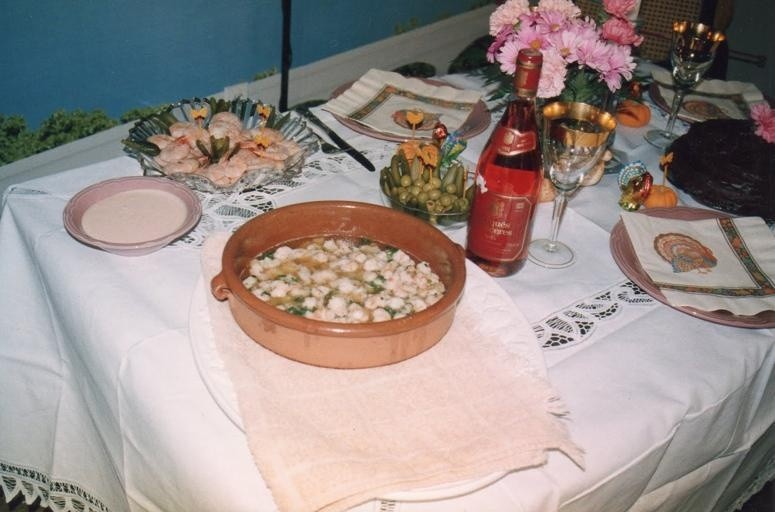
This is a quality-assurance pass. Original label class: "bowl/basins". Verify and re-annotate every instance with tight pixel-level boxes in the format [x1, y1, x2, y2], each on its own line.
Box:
[124, 96, 322, 196]
[659, 133, 775, 219]
[62, 174, 203, 259]
[380, 147, 480, 228]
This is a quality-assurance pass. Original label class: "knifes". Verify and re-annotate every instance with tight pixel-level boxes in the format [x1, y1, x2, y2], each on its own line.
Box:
[295, 104, 376, 174]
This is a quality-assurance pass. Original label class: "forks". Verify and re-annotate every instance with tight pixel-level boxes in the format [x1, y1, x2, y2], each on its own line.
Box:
[312, 130, 353, 155]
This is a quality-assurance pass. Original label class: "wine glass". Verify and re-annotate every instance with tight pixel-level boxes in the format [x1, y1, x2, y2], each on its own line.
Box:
[523, 101, 618, 269]
[645, 18, 725, 151]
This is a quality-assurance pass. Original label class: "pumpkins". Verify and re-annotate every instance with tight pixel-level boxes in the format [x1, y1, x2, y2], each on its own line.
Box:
[615, 99, 650, 127]
[643, 185, 676, 208]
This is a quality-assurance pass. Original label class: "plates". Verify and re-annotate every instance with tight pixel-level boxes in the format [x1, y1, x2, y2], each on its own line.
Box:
[188, 219, 550, 502]
[328, 73, 492, 147]
[610, 205, 775, 329]
[647, 79, 753, 125]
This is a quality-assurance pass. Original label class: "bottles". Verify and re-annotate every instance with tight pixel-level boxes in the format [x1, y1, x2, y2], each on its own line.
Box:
[466, 49, 545, 279]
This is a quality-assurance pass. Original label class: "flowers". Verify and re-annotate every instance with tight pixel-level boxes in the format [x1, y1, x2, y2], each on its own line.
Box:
[483, 2, 648, 156]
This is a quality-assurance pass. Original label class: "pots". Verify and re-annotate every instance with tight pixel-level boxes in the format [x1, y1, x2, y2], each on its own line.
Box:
[210, 200, 466, 369]
